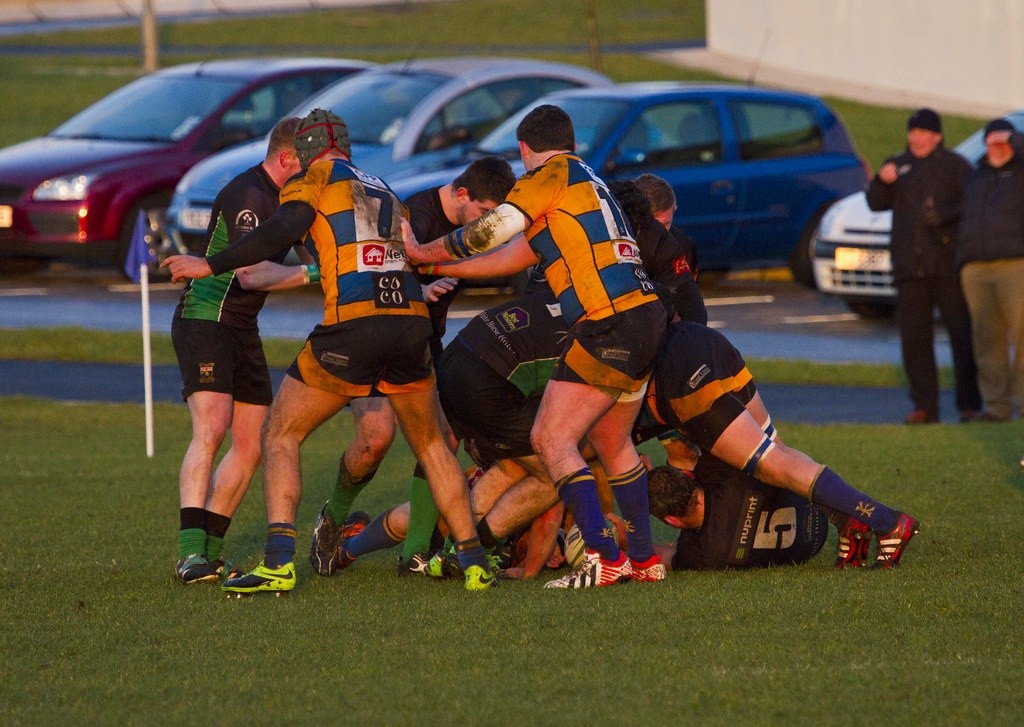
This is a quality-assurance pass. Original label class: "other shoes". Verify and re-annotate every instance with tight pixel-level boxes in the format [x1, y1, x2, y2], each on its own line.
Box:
[210, 559, 244, 581]
[175, 552, 219, 585]
[977, 412, 1010, 424]
[960, 414, 981, 425]
[903, 407, 941, 425]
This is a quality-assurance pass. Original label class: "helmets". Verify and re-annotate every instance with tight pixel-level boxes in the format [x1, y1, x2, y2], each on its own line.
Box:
[293, 107, 352, 167]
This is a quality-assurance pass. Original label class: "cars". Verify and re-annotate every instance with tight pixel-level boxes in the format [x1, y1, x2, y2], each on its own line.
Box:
[372, 79, 867, 291]
[808, 111, 996, 311]
[164, 55, 667, 281]
[0, 58, 378, 277]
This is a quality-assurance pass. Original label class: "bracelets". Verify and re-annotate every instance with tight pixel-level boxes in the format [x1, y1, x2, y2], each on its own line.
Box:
[428, 263, 434, 275]
[301, 265, 320, 285]
[433, 263, 439, 275]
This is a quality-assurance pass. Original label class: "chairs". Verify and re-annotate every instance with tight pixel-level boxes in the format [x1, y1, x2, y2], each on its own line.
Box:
[678, 112, 721, 146]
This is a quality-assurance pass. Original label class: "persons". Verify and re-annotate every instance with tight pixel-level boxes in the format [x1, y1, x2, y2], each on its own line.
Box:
[174, 117, 322, 582]
[866, 109, 974, 424]
[160, 108, 498, 596]
[309, 155, 922, 588]
[954, 119, 1024, 424]
[400, 104, 668, 588]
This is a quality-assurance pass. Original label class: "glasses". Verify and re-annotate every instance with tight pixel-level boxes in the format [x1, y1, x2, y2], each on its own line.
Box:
[985, 140, 1008, 149]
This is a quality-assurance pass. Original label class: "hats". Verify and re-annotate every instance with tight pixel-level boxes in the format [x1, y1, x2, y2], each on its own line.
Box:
[906, 107, 942, 134]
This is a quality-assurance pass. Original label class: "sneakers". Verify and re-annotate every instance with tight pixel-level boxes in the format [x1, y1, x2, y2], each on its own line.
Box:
[309, 499, 338, 576]
[628, 555, 667, 582]
[336, 510, 371, 570]
[220, 559, 297, 597]
[396, 538, 515, 591]
[871, 511, 921, 571]
[831, 513, 870, 569]
[541, 545, 633, 590]
